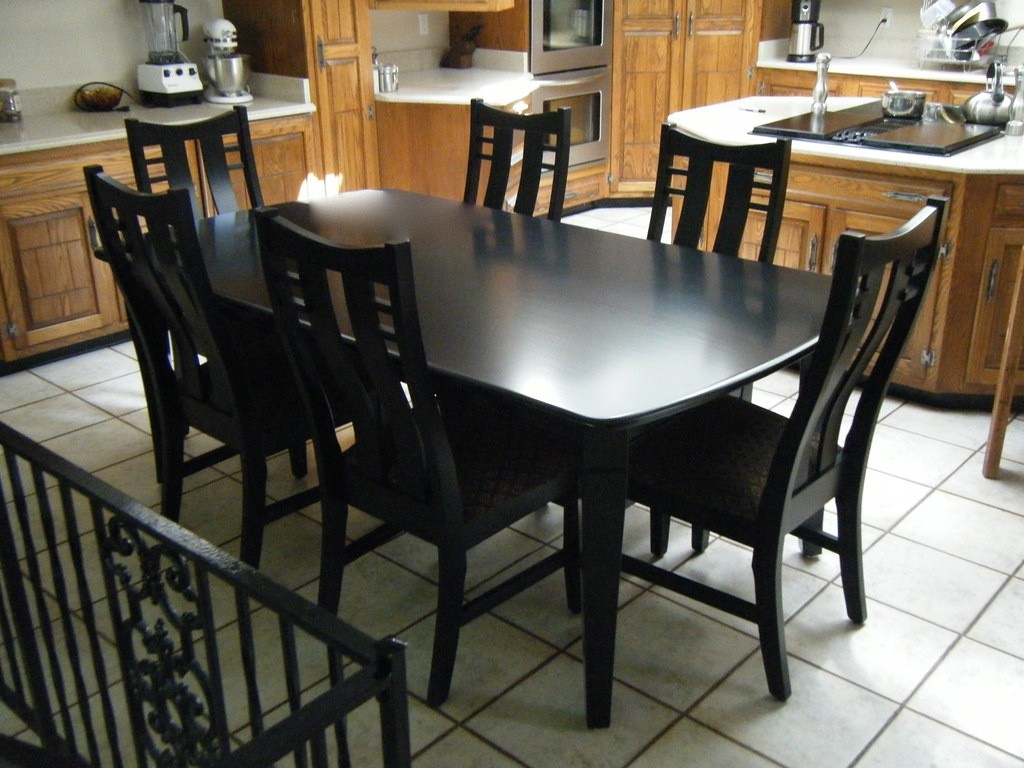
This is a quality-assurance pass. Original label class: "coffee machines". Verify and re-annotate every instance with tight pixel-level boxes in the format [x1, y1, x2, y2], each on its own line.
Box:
[786, 1, 824, 63]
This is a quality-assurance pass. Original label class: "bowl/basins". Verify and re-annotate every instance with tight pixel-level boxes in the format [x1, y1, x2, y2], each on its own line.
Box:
[80, 88, 126, 108]
[205, 54, 255, 98]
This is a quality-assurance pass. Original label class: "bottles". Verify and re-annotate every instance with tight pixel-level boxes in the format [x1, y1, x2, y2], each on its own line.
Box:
[0, 79, 23, 122]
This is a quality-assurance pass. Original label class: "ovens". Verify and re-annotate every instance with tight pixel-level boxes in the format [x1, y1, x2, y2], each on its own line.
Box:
[526, 1, 611, 168]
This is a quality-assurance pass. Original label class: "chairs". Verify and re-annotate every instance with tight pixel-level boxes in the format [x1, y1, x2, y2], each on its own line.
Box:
[82, 162, 354, 572]
[123, 103, 310, 480]
[646, 123, 792, 552]
[462, 96, 571, 222]
[617, 193, 953, 704]
[248, 203, 583, 710]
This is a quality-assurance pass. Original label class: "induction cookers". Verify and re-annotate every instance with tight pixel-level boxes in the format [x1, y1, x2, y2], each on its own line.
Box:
[755, 91, 1002, 155]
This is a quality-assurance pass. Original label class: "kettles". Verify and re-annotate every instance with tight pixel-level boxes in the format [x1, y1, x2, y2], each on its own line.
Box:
[962, 61, 1012, 124]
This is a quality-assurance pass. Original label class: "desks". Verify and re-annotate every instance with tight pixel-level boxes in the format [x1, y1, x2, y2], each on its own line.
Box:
[91, 188, 919, 730]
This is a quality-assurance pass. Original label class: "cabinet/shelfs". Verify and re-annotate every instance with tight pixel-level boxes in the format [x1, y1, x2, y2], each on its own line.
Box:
[608, 0, 792, 209]
[671, 151, 1024, 415]
[372, 65, 539, 213]
[0, 112, 318, 377]
[755, 68, 1017, 109]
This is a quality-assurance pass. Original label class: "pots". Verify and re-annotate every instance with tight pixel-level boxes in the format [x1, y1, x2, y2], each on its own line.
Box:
[881, 81, 926, 119]
[921, 0, 1023, 59]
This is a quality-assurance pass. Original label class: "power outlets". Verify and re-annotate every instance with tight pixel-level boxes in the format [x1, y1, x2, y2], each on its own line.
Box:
[879, 8, 892, 27]
[418, 13, 429, 36]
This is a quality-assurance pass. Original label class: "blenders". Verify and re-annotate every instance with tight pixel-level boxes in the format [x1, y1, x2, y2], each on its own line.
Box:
[199, 18, 254, 103]
[132, 0, 204, 96]
